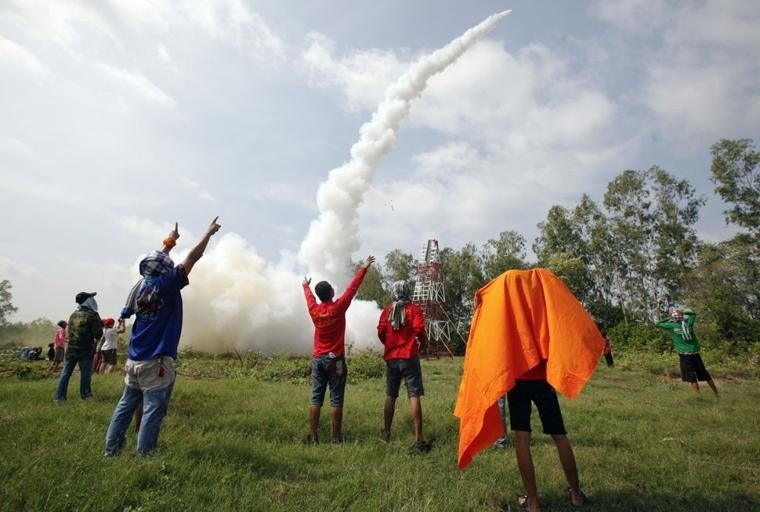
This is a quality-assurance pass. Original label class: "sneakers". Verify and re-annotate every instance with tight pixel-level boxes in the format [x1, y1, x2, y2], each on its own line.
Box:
[303, 437, 318, 444]
[494, 437, 506, 447]
[419, 444, 430, 452]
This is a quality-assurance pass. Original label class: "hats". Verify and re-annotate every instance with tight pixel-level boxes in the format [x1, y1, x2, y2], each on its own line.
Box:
[75, 292, 97, 304]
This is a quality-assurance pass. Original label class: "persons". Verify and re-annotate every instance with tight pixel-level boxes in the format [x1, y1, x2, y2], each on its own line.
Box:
[507, 360, 587, 512]
[104, 216, 222, 460]
[135, 399, 142, 433]
[54, 292, 102, 403]
[92, 320, 107, 373]
[48, 343, 55, 361]
[99, 318, 125, 374]
[602, 331, 614, 365]
[377, 279, 434, 451]
[655, 311, 722, 403]
[47, 320, 70, 373]
[303, 255, 375, 443]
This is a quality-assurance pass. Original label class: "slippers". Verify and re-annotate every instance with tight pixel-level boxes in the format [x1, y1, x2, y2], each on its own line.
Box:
[567, 485, 585, 507]
[518, 495, 528, 511]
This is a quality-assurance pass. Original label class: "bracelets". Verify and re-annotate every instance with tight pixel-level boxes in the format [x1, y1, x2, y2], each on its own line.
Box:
[164, 238, 177, 248]
